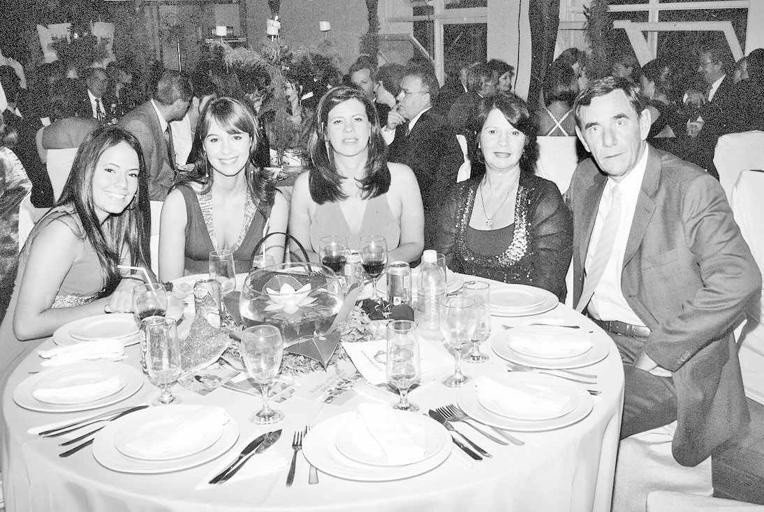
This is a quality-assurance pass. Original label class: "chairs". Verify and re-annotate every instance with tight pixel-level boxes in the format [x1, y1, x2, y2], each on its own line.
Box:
[455, 133, 580, 198]
[643, 489, 762, 512]
[727, 167, 763, 408]
[611, 318, 747, 512]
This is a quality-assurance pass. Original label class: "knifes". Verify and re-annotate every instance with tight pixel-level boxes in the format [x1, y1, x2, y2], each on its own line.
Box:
[40, 405, 151, 460]
[210, 430, 282, 483]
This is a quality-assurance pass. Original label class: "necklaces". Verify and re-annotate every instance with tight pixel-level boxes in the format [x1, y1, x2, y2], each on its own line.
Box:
[480, 176, 519, 227]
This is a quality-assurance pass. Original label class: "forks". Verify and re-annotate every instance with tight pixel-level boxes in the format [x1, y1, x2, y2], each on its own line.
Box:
[284, 428, 304, 483]
[425, 403, 526, 462]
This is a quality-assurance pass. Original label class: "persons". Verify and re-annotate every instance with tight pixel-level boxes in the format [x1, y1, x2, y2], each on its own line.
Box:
[380, 67, 467, 250]
[292, 88, 426, 270]
[160, 95, 289, 285]
[434, 92, 573, 304]
[561, 75, 762, 466]
[0, 126, 157, 410]
[1, 48, 762, 205]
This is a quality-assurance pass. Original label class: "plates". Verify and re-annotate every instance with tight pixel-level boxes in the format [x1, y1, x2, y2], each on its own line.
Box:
[336, 414, 442, 463]
[454, 385, 594, 436]
[487, 282, 549, 312]
[478, 372, 575, 420]
[36, 367, 125, 403]
[52, 313, 143, 351]
[10, 365, 145, 411]
[480, 281, 560, 321]
[300, 417, 455, 479]
[506, 326, 594, 358]
[490, 325, 613, 370]
[112, 410, 224, 462]
[90, 404, 240, 477]
[66, 317, 139, 339]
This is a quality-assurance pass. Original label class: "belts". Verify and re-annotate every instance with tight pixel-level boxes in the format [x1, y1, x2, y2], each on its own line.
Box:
[594, 321, 648, 339]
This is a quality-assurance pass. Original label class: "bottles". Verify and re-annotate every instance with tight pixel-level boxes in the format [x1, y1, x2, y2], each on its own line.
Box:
[109, 96, 118, 124]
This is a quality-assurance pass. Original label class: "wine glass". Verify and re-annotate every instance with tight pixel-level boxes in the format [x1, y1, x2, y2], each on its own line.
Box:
[130, 234, 490, 425]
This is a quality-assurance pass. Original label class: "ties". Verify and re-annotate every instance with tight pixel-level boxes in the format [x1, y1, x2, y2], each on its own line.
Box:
[166, 125, 177, 167]
[705, 83, 712, 101]
[402, 126, 410, 138]
[575, 186, 624, 314]
[95, 98, 100, 116]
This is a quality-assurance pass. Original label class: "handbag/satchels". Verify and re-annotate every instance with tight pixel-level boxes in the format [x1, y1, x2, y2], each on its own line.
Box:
[249, 232, 327, 291]
